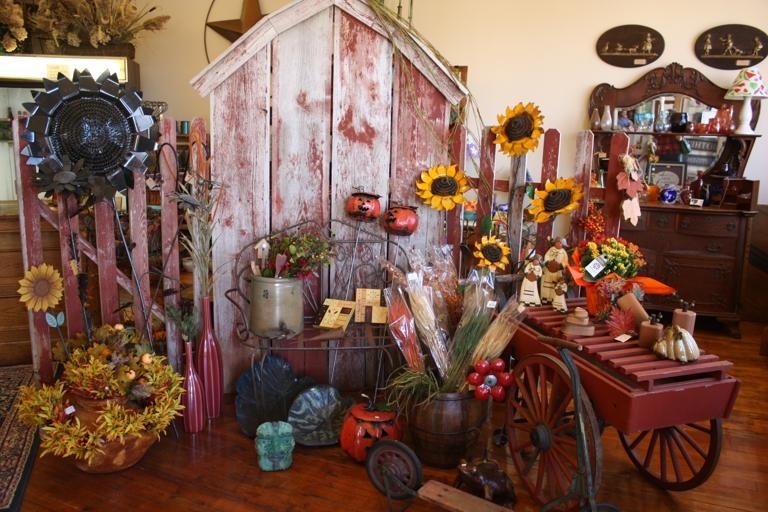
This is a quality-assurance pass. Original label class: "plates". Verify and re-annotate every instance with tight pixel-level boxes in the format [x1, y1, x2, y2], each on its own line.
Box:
[653, 171, 680, 188]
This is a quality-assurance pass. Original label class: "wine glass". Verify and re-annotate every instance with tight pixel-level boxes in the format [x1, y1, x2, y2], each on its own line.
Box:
[670, 99, 736, 134]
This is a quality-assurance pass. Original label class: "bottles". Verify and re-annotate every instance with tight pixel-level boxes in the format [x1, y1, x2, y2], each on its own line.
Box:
[694, 177, 711, 205]
[590, 105, 612, 129]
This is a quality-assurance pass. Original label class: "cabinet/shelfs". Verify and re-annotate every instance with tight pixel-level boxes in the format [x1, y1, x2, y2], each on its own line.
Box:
[589, 63, 761, 339]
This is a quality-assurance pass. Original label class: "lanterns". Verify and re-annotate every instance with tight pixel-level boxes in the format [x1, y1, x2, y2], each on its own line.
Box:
[339, 402, 402, 465]
[346, 192, 381, 222]
[382, 206, 417, 236]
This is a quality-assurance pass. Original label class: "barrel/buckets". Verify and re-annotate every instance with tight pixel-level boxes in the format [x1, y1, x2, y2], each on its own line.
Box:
[408, 390, 489, 469]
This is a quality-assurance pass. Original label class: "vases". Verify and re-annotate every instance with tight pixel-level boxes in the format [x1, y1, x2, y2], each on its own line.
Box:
[197, 297, 223, 420]
[249, 274, 304, 340]
[182, 341, 206, 435]
[585, 283, 607, 316]
[69, 396, 156, 475]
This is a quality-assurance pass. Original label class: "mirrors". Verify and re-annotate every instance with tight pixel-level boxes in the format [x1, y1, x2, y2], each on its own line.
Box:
[613, 93, 727, 183]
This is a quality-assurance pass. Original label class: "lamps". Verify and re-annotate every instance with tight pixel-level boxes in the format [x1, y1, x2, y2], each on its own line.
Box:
[723, 67, 768, 136]
[0, 53, 128, 84]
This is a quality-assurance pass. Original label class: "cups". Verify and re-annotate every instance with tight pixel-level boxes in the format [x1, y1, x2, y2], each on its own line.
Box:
[181, 121, 188, 135]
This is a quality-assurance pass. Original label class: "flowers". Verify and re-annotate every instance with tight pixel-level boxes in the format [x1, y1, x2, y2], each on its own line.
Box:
[581, 237, 647, 278]
[14, 263, 187, 467]
[528, 176, 586, 223]
[262, 223, 338, 279]
[0, 0, 171, 53]
[415, 163, 470, 211]
[473, 234, 512, 271]
[491, 101, 544, 157]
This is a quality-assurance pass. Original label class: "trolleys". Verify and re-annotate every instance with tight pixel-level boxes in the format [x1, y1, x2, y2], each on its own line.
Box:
[460, 265, 737, 507]
